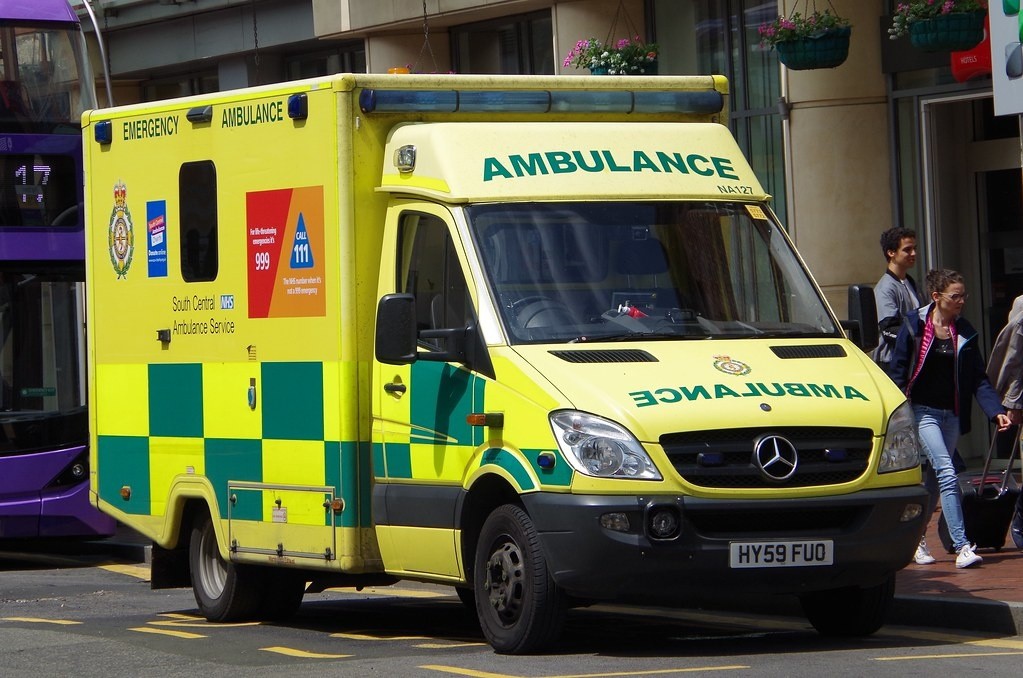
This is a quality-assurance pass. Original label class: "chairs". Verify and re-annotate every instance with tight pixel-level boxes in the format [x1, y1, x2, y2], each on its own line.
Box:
[429, 250, 467, 358]
[614, 234, 686, 309]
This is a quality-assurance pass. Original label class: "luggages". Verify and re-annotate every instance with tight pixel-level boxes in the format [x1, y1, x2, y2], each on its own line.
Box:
[937, 422, 1020, 551]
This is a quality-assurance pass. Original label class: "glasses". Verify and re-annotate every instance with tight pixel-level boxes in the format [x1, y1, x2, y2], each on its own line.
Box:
[939, 292, 970, 302]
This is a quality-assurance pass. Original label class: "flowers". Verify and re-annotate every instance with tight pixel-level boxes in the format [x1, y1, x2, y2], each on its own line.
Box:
[887, 0, 989, 41]
[759, 9, 854, 52]
[562, 35, 662, 77]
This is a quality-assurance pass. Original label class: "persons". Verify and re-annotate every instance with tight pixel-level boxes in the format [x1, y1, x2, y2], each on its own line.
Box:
[871, 226, 929, 376]
[887, 268, 1012, 569]
[985, 295, 1023, 550]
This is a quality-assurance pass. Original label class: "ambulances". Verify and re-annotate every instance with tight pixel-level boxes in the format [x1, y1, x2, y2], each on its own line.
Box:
[87, 66, 925, 658]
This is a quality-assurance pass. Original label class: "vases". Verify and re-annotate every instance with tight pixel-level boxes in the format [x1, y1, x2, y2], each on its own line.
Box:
[589, 63, 658, 75]
[909, 12, 987, 52]
[776, 37, 850, 71]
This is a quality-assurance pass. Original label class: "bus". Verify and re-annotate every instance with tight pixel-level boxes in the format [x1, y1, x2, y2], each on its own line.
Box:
[2, 1, 112, 548]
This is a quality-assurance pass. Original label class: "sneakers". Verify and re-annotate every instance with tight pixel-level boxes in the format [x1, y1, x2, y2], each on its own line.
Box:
[956, 544, 983, 569]
[912, 540, 936, 565]
